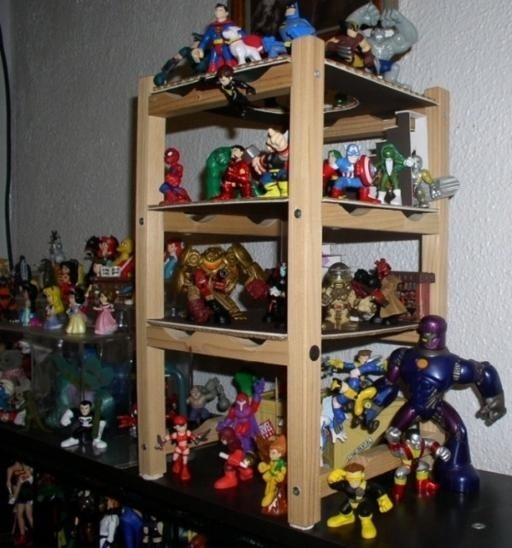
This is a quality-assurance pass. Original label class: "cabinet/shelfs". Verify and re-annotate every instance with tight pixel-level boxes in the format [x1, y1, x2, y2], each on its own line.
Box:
[0, 36, 511, 548]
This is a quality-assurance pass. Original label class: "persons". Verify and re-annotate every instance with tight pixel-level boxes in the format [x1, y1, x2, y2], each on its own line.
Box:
[0, 0, 507, 548]
